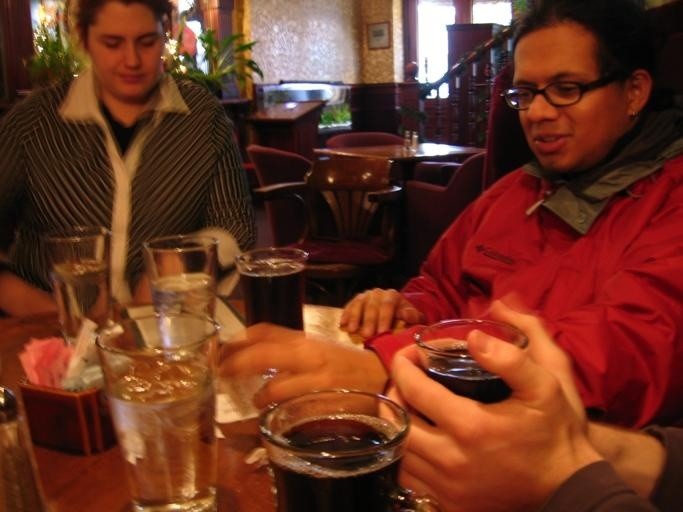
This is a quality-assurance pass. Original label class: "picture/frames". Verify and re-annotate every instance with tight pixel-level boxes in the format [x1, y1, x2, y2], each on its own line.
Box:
[366, 21, 391, 50]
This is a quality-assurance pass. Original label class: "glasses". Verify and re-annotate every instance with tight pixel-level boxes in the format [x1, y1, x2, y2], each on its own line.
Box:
[502, 74, 613, 111]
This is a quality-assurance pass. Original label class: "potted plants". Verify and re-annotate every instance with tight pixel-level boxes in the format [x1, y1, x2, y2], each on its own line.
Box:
[169, 30, 264, 128]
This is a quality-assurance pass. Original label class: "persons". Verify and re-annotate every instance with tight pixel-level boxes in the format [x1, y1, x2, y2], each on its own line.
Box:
[215, 0, 682, 432]
[2, 2, 259, 316]
[379, 299, 682, 508]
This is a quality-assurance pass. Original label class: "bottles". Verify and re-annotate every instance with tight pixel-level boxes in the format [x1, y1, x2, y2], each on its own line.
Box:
[404, 131, 419, 152]
[0, 386, 51, 512]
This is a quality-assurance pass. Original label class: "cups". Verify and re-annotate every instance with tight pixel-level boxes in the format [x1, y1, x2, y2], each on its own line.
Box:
[231, 246, 310, 331]
[38, 225, 113, 337]
[256, 387, 411, 512]
[138, 234, 220, 358]
[412, 318, 530, 404]
[96, 312, 222, 512]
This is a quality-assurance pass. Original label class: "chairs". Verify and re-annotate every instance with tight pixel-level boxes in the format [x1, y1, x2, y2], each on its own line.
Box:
[239, 141, 312, 242]
[406, 149, 488, 235]
[312, 147, 405, 245]
[324, 132, 409, 149]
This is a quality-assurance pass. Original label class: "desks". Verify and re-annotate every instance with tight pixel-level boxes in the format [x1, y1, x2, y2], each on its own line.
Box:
[1, 299, 400, 511]
[315, 142, 479, 244]
